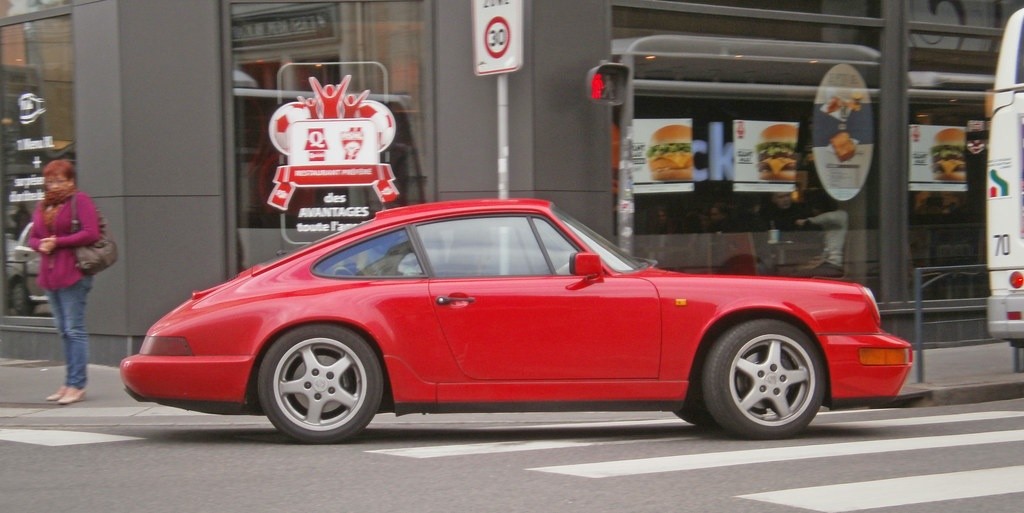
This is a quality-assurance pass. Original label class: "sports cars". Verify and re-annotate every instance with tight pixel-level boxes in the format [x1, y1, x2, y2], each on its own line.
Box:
[119, 197, 914, 445]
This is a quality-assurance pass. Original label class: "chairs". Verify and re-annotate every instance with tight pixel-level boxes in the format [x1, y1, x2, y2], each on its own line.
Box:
[685, 229, 759, 273]
[906, 223, 989, 299]
[811, 234, 851, 280]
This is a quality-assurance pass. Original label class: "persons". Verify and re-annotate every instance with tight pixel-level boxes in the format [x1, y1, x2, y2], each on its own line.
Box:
[758, 189, 849, 277]
[646, 202, 755, 276]
[29, 159, 102, 404]
[11, 202, 32, 240]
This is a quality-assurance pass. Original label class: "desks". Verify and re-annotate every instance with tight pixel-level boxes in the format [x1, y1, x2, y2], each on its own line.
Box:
[761, 240, 814, 273]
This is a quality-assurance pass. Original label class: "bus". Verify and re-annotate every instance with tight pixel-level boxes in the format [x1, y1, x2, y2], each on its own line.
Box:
[984, 6, 1024, 350]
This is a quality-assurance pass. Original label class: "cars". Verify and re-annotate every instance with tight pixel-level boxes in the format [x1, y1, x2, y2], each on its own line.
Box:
[4, 221, 52, 316]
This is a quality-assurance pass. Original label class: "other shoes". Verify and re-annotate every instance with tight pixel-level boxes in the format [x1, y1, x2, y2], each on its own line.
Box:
[57, 388, 86, 404]
[45, 391, 63, 401]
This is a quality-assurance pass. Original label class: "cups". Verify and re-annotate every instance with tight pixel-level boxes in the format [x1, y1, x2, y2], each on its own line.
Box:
[768, 230, 781, 243]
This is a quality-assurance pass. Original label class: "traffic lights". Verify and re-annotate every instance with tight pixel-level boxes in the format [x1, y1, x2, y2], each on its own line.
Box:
[584, 60, 630, 107]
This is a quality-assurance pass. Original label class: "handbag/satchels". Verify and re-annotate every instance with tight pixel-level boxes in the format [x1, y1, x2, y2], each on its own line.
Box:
[73, 190, 118, 276]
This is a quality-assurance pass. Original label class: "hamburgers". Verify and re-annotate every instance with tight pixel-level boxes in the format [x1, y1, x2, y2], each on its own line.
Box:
[644, 124, 692, 179]
[931, 127, 968, 181]
[754, 124, 800, 182]
